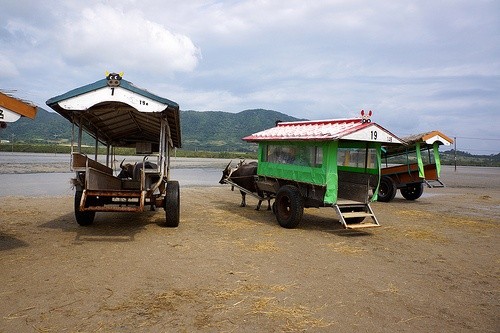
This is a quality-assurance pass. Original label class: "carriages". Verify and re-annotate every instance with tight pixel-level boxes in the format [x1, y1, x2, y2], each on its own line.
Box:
[219, 110, 382, 231]
[45, 72, 183, 226]
[345, 132, 451, 203]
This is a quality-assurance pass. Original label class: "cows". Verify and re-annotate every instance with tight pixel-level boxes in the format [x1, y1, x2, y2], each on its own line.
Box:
[218, 158, 279, 211]
[116, 158, 159, 211]
